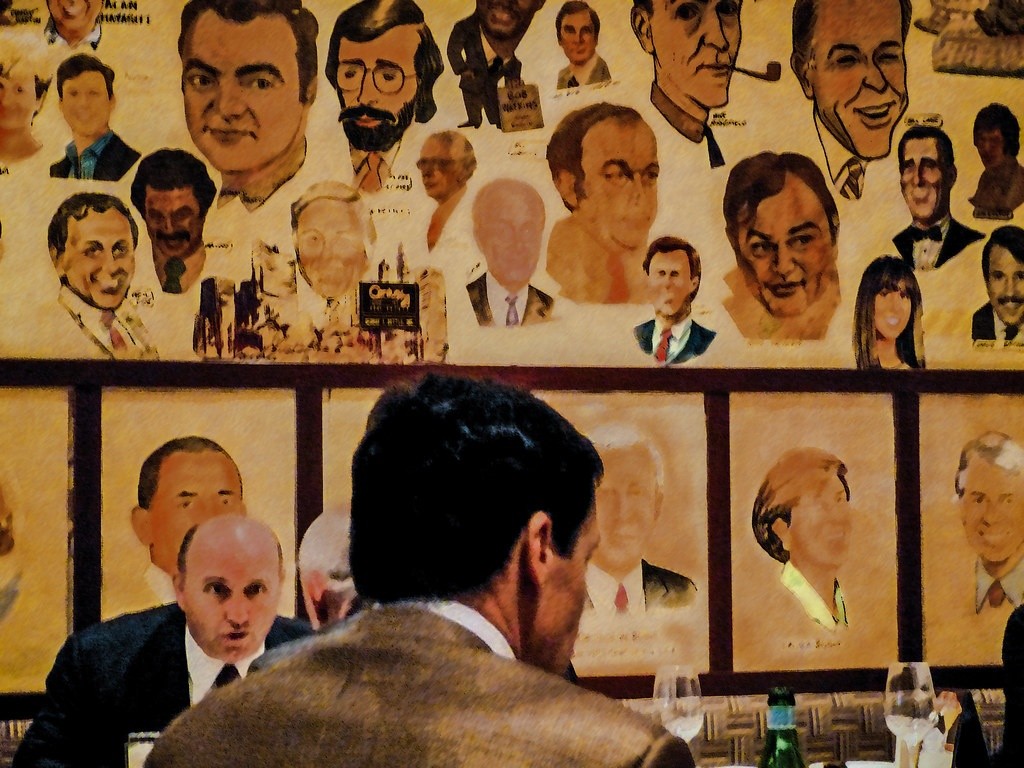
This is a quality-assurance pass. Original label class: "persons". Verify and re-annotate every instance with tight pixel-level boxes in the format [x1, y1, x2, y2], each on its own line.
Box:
[11, 512, 306, 768]
[142, 374, 694, 767]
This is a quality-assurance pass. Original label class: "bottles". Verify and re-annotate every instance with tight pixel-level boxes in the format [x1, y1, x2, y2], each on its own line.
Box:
[757, 686, 806, 768]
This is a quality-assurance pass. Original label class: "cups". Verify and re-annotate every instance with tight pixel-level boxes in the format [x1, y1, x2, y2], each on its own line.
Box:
[126, 732, 159, 767]
[650, 665, 703, 739]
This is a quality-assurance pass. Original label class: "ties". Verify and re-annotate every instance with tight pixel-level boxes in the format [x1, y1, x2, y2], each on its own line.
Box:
[216, 662, 237, 688]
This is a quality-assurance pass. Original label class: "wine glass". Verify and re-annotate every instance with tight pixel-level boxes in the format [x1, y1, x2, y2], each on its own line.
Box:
[884, 662, 938, 768]
